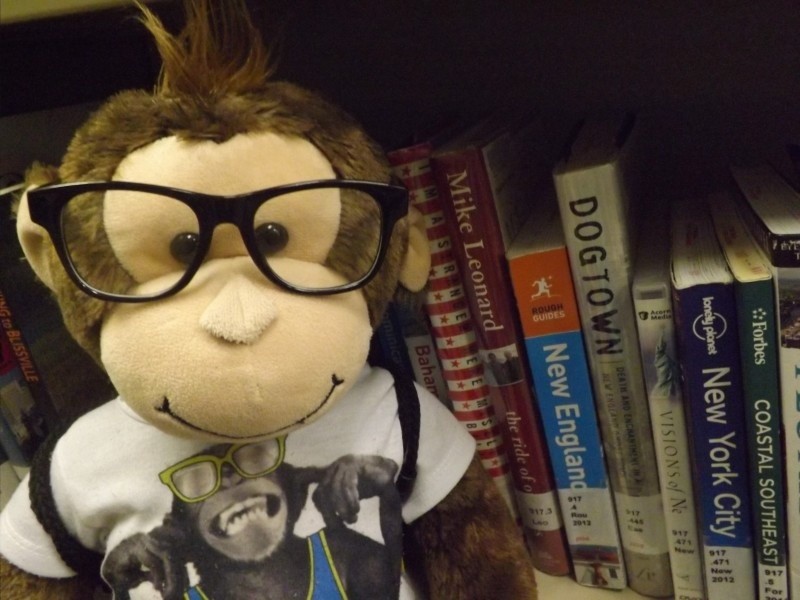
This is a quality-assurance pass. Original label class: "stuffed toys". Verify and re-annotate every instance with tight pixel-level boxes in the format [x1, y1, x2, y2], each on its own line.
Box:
[0, 0, 538, 599]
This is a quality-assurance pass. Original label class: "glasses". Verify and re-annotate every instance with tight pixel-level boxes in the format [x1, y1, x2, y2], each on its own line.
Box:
[25, 172, 411, 302]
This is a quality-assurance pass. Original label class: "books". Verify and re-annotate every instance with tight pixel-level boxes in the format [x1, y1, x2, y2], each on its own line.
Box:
[0, 122, 800, 600]
